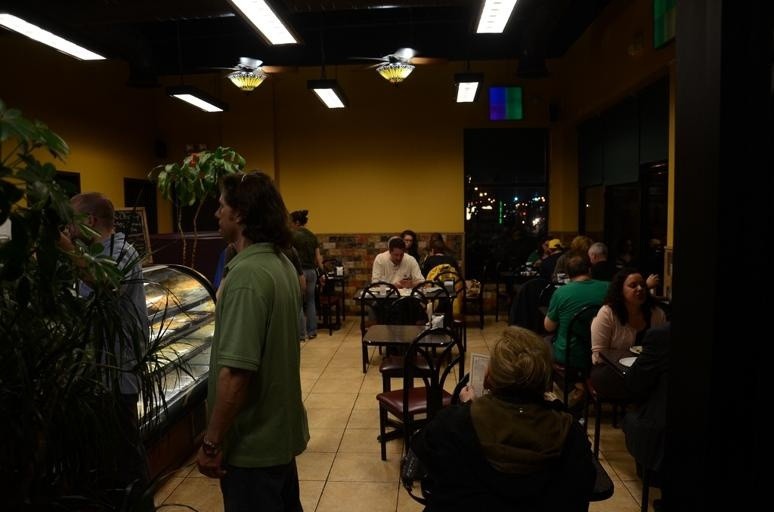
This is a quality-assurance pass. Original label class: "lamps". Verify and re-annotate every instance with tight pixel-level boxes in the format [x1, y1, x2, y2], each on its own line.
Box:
[308, 79, 350, 109]
[451, 72, 482, 103]
[231, 0, 297, 45]
[0, 12, 107, 63]
[227, 71, 267, 91]
[376, 62, 416, 83]
[474, 0, 519, 35]
[173, 93, 223, 113]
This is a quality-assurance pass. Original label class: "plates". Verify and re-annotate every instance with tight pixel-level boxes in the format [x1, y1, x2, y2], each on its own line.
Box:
[391, 288, 419, 296]
[619, 358, 638, 367]
[367, 284, 393, 292]
[630, 345, 643, 354]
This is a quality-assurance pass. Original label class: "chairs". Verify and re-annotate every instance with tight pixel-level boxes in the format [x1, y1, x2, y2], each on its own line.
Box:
[582, 364, 619, 456]
[360, 272, 401, 374]
[467, 262, 500, 329]
[376, 327, 465, 461]
[434, 272, 466, 323]
[551, 304, 599, 408]
[411, 281, 453, 363]
[316, 258, 347, 336]
[381, 298, 434, 391]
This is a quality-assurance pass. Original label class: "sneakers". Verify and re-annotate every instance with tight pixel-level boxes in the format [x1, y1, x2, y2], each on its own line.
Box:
[300, 339, 305, 342]
[308, 334, 316, 339]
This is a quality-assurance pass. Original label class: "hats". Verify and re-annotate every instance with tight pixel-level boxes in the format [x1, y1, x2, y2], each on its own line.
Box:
[549, 239, 562, 249]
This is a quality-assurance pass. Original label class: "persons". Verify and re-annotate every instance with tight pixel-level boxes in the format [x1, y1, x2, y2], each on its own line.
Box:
[588, 242, 615, 281]
[371, 238, 426, 289]
[613, 236, 662, 288]
[539, 238, 565, 279]
[8, 191, 154, 512]
[411, 325, 616, 512]
[526, 236, 551, 274]
[216, 240, 307, 298]
[552, 236, 594, 286]
[401, 230, 420, 262]
[421, 240, 466, 281]
[590, 267, 668, 366]
[194, 171, 312, 512]
[289, 209, 324, 343]
[430, 233, 457, 255]
[543, 254, 612, 367]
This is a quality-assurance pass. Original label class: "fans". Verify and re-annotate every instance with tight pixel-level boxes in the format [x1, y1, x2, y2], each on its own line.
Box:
[202, 57, 292, 79]
[349, 47, 434, 70]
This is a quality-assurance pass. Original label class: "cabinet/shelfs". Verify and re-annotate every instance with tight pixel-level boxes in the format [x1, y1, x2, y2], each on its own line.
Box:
[134, 263, 217, 501]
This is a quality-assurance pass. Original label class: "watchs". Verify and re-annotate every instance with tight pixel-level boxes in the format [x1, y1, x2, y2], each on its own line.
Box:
[201, 437, 222, 457]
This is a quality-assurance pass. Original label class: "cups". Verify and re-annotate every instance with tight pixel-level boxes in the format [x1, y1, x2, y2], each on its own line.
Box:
[379, 286, 386, 295]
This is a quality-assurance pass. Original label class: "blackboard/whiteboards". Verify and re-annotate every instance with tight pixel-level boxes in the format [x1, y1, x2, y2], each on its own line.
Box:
[113, 205, 153, 265]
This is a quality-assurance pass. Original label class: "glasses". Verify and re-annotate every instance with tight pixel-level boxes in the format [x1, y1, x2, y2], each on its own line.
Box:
[71, 213, 91, 220]
[240, 169, 255, 183]
